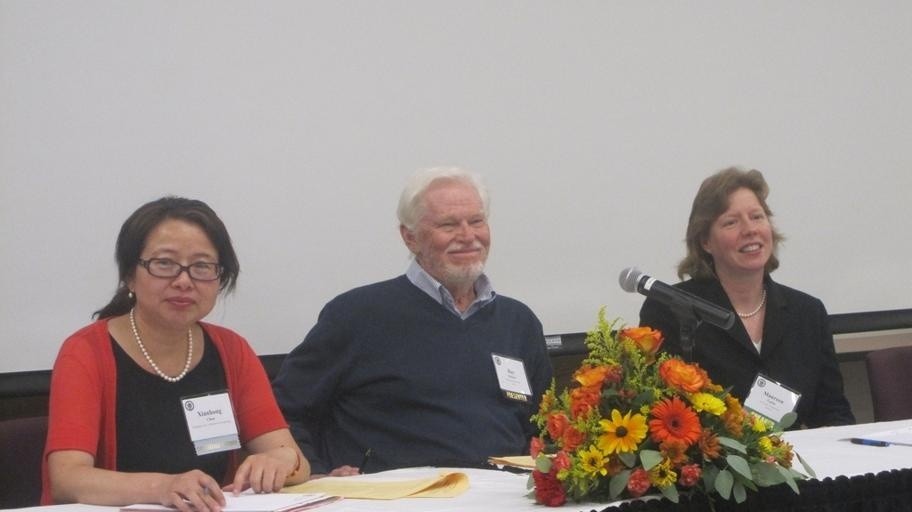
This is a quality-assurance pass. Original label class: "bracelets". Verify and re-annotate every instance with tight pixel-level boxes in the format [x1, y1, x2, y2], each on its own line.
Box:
[277, 443, 301, 479]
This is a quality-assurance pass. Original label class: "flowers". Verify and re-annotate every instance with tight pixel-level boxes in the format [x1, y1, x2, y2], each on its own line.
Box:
[523, 302, 816, 510]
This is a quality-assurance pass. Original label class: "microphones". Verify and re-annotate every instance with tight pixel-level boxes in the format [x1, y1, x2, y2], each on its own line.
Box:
[619, 267, 735, 330]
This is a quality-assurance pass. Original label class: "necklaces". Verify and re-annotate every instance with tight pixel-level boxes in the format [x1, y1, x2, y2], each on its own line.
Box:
[734, 285, 768, 319]
[129, 303, 194, 384]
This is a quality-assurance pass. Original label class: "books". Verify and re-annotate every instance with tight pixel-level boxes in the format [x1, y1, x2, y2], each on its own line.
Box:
[119, 489, 343, 512]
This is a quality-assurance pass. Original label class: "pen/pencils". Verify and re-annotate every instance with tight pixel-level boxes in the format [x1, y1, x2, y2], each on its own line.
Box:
[201, 486, 208, 494]
[358, 447, 373, 474]
[851, 436, 889, 447]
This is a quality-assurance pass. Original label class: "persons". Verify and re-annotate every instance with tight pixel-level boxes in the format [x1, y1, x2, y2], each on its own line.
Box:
[38, 196, 313, 512]
[268, 165, 556, 478]
[637, 165, 859, 431]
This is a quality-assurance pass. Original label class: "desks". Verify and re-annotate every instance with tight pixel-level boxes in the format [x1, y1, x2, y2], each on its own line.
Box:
[3, 418, 911, 512]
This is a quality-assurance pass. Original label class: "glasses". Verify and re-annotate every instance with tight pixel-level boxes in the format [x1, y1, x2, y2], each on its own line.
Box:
[130, 255, 225, 282]
